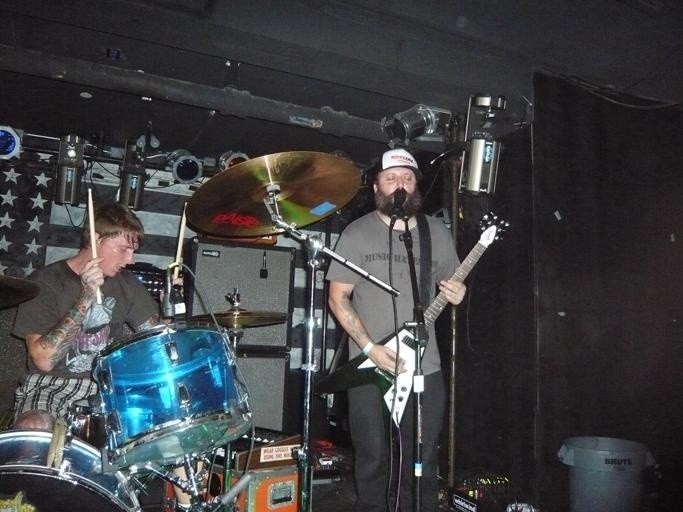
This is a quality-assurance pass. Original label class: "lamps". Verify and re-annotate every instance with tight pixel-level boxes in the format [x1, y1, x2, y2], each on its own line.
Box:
[0, 122, 251, 208]
[377, 92, 507, 199]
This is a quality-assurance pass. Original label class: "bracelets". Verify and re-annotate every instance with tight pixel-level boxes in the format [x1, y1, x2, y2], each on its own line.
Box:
[361, 338, 379, 355]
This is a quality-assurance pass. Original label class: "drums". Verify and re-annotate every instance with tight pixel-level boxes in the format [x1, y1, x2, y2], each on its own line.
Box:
[0, 430, 144, 512]
[66, 398, 116, 441]
[91, 321, 254, 471]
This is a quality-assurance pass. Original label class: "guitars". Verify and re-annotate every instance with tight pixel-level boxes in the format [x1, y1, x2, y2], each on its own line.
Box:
[314, 211, 511, 448]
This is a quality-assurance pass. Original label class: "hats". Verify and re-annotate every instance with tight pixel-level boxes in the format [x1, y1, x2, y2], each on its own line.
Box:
[374, 149, 422, 182]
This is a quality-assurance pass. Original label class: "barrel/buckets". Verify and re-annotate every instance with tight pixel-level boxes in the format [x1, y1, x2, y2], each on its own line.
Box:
[555, 436, 658, 512]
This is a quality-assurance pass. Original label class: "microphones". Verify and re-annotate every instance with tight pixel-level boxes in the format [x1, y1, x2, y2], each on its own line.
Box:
[391, 190, 407, 219]
[159, 271, 175, 316]
[259, 252, 268, 277]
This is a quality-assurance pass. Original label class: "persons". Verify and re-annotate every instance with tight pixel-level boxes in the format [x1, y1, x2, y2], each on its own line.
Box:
[324, 147, 467, 510]
[6, 202, 209, 512]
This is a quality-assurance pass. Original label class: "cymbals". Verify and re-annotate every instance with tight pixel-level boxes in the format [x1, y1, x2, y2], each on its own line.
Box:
[186, 151, 361, 239]
[0, 276, 40, 310]
[188, 309, 287, 329]
